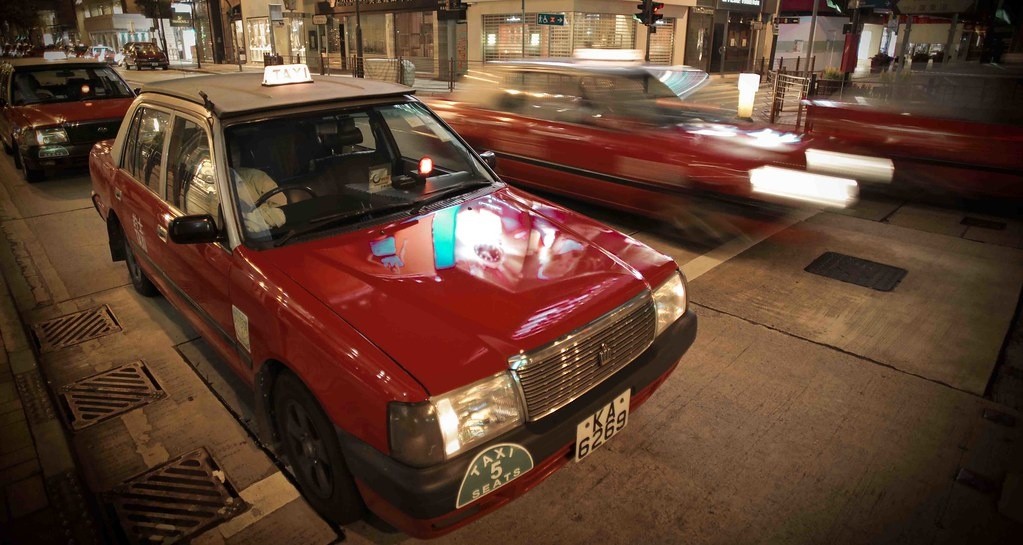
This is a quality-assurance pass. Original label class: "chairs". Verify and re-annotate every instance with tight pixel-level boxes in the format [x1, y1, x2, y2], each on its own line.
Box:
[285, 121, 374, 206]
[65, 77, 94, 100]
[14, 75, 42, 102]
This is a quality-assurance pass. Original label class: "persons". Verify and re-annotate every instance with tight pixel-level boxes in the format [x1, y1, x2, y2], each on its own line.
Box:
[14, 74, 55, 102]
[185, 132, 288, 236]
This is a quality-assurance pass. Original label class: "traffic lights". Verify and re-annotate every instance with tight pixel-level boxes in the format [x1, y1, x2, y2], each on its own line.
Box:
[636, 0, 664, 26]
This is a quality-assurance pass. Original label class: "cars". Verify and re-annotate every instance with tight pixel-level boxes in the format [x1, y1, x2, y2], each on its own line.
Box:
[0, 58, 141, 183]
[90, 62, 700, 539]
[803, 68, 1023, 216]
[436, 46, 859, 257]
[0, 41, 89, 59]
[83, 45, 119, 67]
[121, 41, 169, 71]
